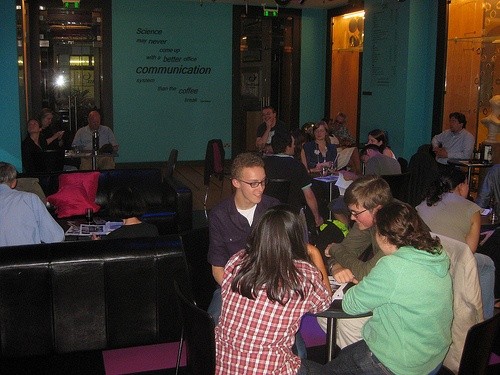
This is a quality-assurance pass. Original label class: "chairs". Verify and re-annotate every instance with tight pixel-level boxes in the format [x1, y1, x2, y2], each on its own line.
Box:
[161, 138, 500, 375]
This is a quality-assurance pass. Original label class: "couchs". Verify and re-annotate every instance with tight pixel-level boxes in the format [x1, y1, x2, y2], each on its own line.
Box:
[17, 167, 193, 234]
[0, 235, 194, 361]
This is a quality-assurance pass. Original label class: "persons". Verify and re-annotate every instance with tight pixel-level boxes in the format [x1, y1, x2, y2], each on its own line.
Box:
[343, 144, 401, 182]
[0, 162, 65, 247]
[255, 106, 285, 154]
[415, 165, 495, 320]
[315, 203, 454, 375]
[301, 121, 337, 176]
[320, 112, 362, 175]
[476, 164, 500, 308]
[207, 152, 282, 327]
[431, 113, 475, 175]
[367, 129, 398, 161]
[91, 190, 159, 240]
[262, 130, 325, 230]
[22, 107, 78, 173]
[215, 205, 337, 375]
[294, 122, 315, 147]
[325, 175, 432, 280]
[72, 111, 118, 170]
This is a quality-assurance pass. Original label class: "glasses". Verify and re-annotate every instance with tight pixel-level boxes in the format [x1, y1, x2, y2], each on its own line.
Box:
[238, 177, 268, 188]
[351, 209, 368, 218]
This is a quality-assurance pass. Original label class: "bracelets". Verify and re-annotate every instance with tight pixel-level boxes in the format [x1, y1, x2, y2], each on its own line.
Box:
[309, 169, 311, 174]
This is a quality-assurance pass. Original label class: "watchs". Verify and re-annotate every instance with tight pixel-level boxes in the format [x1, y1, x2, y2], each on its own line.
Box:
[327, 261, 336, 274]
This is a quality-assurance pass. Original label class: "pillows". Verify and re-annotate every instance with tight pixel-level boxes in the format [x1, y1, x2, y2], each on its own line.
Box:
[58, 171, 101, 201]
[47, 181, 100, 219]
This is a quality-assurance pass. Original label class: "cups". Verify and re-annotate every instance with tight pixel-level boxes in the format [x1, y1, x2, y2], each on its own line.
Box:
[320, 165, 328, 176]
[85, 208, 93, 222]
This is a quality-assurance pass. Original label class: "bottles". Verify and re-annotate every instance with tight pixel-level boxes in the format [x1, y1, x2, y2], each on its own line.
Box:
[92, 129, 99, 151]
[472, 144, 477, 162]
[477, 144, 483, 162]
[488, 146, 492, 163]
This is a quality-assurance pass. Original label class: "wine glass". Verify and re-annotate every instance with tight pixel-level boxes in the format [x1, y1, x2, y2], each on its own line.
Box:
[328, 162, 336, 176]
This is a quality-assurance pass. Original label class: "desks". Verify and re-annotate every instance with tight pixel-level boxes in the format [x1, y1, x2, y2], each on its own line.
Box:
[313, 173, 339, 220]
[60, 216, 158, 237]
[65, 150, 119, 170]
[307, 283, 374, 361]
[447, 158, 493, 187]
